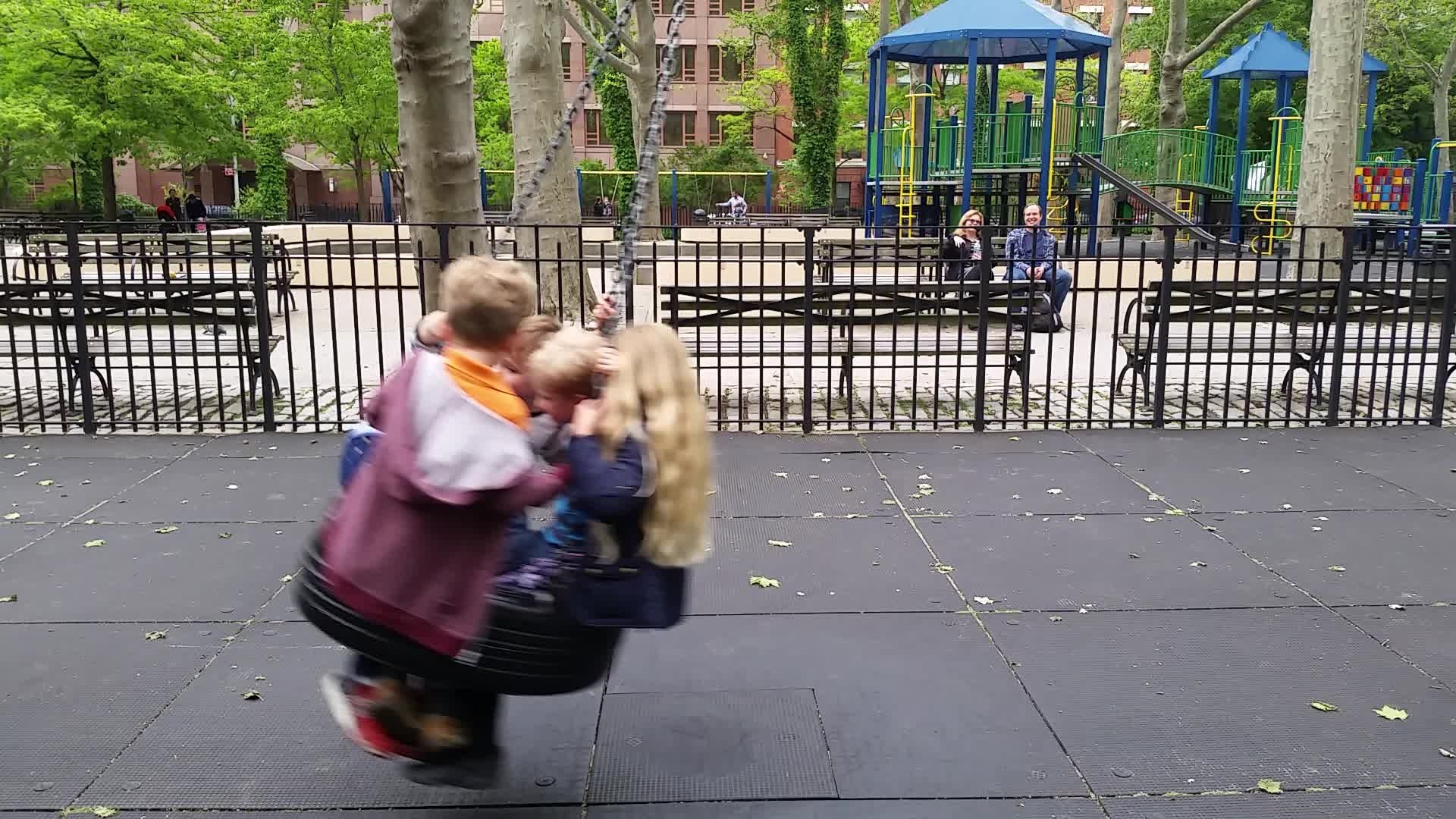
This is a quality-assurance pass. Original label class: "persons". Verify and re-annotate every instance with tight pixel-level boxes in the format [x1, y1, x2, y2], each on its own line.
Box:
[714, 189, 748, 223]
[943, 209, 998, 317]
[1004, 203, 1073, 331]
[156, 189, 207, 233]
[290, 255, 719, 789]
[593, 197, 612, 217]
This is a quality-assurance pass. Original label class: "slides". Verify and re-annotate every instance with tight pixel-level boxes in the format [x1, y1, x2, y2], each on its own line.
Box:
[1070, 152, 1250, 253]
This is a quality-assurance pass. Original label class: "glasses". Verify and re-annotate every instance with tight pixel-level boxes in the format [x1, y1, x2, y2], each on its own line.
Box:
[968, 219, 981, 225]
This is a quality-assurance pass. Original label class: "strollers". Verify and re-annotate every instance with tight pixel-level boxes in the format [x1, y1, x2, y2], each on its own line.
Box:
[156, 205, 183, 234]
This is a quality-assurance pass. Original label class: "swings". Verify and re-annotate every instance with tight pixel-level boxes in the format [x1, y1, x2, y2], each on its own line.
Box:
[341, 0, 687, 697]
[694, 175, 715, 215]
[598, 174, 620, 208]
[728, 175, 748, 208]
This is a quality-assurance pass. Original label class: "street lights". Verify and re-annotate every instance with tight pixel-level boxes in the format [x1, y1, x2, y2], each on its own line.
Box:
[70, 160, 78, 207]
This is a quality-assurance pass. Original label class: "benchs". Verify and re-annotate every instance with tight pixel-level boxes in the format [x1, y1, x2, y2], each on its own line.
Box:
[24, 234, 299, 316]
[484, 211, 512, 224]
[581, 216, 618, 228]
[661, 279, 1047, 414]
[818, 237, 1006, 330]
[0, 279, 285, 416]
[707, 212, 862, 227]
[1, 216, 245, 243]
[1112, 278, 1456, 410]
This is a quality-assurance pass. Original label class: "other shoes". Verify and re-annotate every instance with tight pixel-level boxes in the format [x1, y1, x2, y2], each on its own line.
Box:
[408, 743, 498, 790]
[320, 669, 407, 763]
[969, 323, 978, 329]
[1012, 322, 1023, 331]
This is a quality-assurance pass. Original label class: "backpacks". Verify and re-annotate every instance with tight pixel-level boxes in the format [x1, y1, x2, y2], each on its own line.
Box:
[1021, 292, 1063, 333]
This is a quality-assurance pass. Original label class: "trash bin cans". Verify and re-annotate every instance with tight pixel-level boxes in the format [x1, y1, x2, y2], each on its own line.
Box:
[1116, 200, 1134, 236]
[120, 211, 133, 233]
[691, 209, 709, 225]
